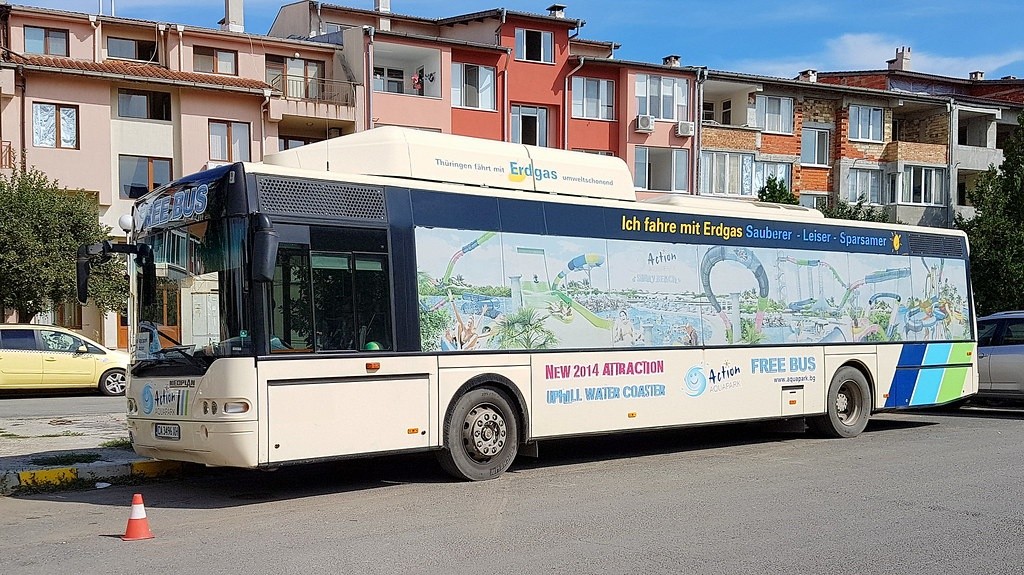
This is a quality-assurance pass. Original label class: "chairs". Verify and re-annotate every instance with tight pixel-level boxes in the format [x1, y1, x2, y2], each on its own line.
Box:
[320, 304, 392, 350]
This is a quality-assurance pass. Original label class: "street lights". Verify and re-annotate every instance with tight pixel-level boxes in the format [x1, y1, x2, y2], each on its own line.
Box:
[119, 214, 132, 279]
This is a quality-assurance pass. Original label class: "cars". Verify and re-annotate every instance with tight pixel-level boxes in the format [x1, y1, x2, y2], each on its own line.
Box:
[0, 322, 131, 396]
[976, 311, 1024, 398]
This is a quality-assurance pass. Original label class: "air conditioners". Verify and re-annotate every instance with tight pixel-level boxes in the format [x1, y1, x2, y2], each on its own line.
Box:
[674, 121, 695, 137]
[634, 115, 655, 133]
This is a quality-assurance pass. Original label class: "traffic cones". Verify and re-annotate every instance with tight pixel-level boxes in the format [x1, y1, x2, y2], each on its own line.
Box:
[119, 493, 157, 542]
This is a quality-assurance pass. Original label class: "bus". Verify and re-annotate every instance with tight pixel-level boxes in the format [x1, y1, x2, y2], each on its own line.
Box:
[74, 127, 981, 484]
